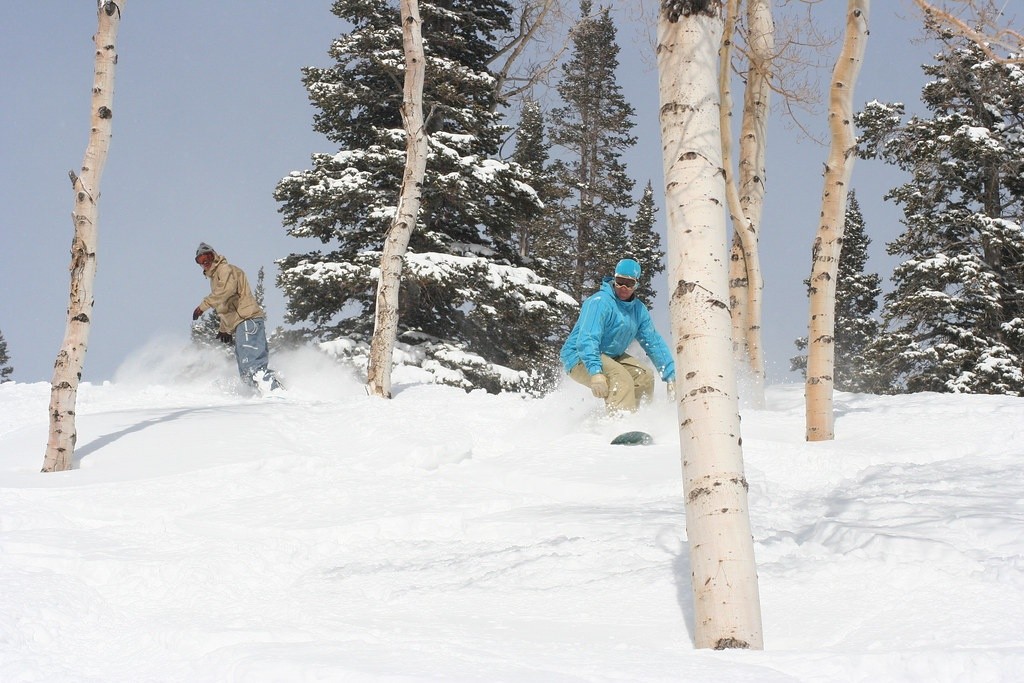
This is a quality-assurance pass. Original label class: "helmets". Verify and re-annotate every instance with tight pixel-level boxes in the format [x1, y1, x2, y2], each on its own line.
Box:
[614, 259, 641, 281]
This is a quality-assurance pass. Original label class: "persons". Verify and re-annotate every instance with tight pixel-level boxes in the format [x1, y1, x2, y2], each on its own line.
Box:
[560, 259, 677, 420]
[193, 243, 285, 393]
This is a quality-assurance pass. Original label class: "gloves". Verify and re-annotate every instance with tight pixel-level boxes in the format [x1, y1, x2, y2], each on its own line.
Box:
[591, 374, 608, 398]
[216, 332, 233, 343]
[192, 307, 203, 320]
[667, 380, 676, 399]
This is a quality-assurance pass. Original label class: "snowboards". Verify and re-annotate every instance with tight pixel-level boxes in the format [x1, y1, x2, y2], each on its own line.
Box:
[611, 430, 655, 446]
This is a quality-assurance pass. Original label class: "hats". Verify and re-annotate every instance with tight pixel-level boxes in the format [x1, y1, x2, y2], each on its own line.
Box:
[194, 242, 214, 259]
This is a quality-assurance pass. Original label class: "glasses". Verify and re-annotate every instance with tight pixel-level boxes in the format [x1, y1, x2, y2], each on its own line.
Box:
[196, 253, 214, 264]
[614, 276, 638, 290]
